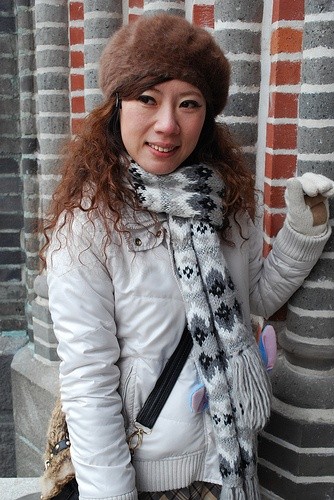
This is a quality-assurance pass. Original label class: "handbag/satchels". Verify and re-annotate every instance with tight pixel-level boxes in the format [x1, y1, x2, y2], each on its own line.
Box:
[41, 402, 79, 499]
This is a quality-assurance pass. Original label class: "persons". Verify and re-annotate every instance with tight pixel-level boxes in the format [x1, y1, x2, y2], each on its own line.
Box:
[32, 7, 334, 500]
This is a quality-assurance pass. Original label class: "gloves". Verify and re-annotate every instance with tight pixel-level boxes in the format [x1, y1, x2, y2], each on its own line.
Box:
[284, 172, 334, 238]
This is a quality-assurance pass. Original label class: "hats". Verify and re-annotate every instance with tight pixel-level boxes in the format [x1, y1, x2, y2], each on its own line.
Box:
[98, 11, 231, 118]
